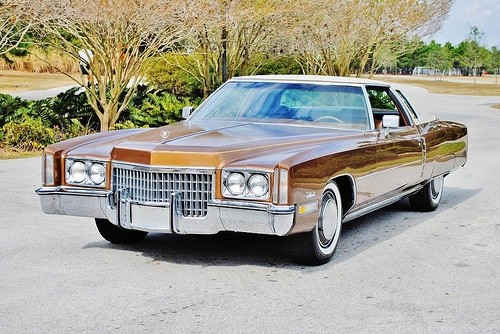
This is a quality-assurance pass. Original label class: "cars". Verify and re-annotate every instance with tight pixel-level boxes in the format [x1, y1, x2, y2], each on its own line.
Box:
[34, 72, 469, 267]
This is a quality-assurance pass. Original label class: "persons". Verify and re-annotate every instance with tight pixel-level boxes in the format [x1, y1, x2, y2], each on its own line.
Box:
[111, 43, 126, 75]
[78, 45, 93, 89]
